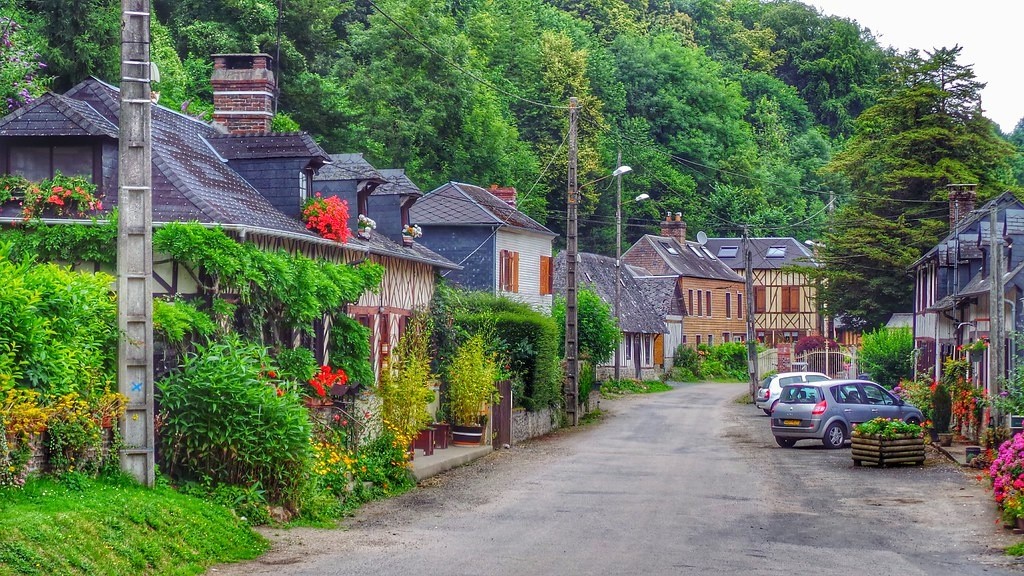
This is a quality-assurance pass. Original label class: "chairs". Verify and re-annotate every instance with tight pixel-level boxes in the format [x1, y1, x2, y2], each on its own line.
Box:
[845, 391, 861, 403]
[797, 391, 812, 403]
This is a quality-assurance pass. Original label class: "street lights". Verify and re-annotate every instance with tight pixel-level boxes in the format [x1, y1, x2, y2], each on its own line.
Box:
[564, 165, 633, 426]
[615, 194, 649, 382]
[805, 240, 834, 339]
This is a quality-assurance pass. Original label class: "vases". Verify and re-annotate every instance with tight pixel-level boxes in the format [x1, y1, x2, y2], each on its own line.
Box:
[358, 228, 365, 235]
[403, 235, 413, 242]
[1015, 515, 1024, 529]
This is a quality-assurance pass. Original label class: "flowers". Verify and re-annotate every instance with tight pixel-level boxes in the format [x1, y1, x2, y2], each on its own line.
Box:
[357, 214, 376, 239]
[989, 420, 1024, 529]
[303, 192, 352, 243]
[0, 172, 104, 225]
[402, 224, 423, 238]
[852, 418, 925, 438]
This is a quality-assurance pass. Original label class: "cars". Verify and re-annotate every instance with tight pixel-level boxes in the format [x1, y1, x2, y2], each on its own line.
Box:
[771, 380, 926, 449]
[755, 372, 861, 416]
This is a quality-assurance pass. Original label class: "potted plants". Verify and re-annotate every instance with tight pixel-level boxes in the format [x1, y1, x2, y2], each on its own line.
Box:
[444, 332, 504, 447]
[932, 383, 954, 446]
